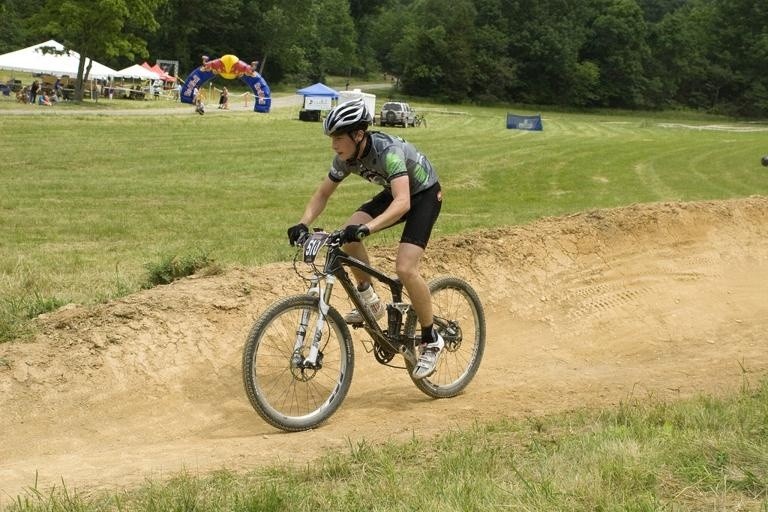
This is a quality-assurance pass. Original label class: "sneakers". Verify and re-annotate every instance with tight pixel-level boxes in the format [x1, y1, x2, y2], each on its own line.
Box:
[413, 334, 444, 379]
[347, 302, 384, 323]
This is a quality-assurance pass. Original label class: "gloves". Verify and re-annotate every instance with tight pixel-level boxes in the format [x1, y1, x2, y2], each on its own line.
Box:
[344, 224, 371, 241]
[286, 225, 308, 246]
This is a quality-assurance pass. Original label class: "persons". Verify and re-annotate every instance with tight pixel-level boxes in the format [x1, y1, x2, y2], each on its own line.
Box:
[218, 88, 226, 109]
[54, 78, 64, 98]
[28, 79, 42, 104]
[173, 81, 183, 102]
[285, 97, 446, 381]
[37, 91, 52, 107]
[48, 90, 59, 103]
[152, 80, 161, 102]
[221, 86, 229, 111]
[192, 85, 200, 106]
[18, 86, 30, 105]
[195, 98, 205, 116]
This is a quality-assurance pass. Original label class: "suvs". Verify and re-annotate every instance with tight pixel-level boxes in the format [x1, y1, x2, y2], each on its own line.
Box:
[379, 102, 417, 129]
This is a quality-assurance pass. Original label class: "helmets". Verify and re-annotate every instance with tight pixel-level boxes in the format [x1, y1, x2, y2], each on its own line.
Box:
[323, 99, 372, 137]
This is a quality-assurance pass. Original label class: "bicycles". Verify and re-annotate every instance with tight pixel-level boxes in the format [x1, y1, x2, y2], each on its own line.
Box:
[416, 113, 427, 128]
[238, 226, 488, 432]
